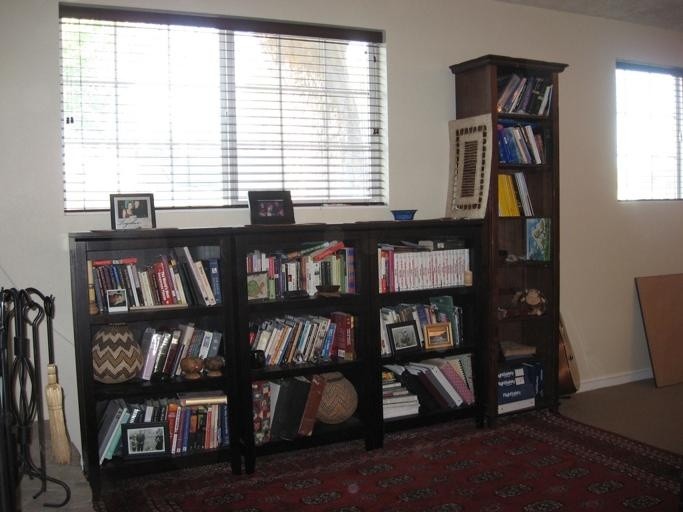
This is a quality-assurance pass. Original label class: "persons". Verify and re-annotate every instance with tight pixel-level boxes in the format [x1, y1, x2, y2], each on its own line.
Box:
[256, 201, 288, 217]
[121, 201, 134, 219]
[131, 200, 148, 217]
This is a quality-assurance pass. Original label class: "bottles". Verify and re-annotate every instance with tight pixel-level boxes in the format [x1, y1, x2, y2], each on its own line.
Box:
[90, 322, 145, 384]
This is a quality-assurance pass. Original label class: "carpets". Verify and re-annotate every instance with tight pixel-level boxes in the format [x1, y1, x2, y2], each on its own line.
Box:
[96, 411, 682, 512]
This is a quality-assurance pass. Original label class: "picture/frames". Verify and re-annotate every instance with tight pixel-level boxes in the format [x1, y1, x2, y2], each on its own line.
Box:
[110, 193, 156, 229]
[248, 190, 294, 224]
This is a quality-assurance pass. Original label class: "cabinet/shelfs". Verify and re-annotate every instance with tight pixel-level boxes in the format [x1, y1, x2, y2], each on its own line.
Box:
[67, 227, 239, 499]
[449, 54, 569, 429]
[234, 218, 486, 477]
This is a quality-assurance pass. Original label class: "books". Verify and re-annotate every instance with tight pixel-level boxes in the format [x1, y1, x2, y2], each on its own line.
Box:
[244, 241, 360, 448]
[85, 246, 225, 316]
[497, 337, 548, 415]
[375, 235, 476, 423]
[497, 70, 555, 165]
[134, 321, 223, 381]
[496, 171, 552, 262]
[98, 389, 233, 466]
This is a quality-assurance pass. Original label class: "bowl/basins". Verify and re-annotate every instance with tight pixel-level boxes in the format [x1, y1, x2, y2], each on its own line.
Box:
[389, 209, 417, 220]
